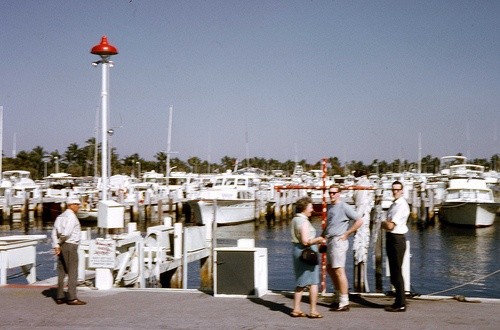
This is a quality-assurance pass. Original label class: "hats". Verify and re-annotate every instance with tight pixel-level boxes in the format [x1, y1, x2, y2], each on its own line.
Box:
[65, 195, 81, 205]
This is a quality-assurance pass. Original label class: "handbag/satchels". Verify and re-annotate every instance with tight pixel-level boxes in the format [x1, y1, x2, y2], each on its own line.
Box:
[299, 243, 319, 265]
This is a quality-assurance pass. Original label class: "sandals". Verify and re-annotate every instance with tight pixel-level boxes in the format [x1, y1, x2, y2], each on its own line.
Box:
[291, 311, 308, 317]
[307, 312, 323, 318]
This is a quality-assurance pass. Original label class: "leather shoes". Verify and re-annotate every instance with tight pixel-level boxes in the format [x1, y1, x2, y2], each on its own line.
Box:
[57, 298, 68, 304]
[67, 299, 86, 305]
[385, 303, 406, 312]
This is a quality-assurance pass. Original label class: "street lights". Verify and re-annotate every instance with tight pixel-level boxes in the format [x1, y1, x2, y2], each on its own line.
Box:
[89, 36, 121, 202]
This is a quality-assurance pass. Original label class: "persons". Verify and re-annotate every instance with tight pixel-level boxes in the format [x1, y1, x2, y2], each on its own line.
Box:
[52, 197, 86, 304]
[381, 181, 410, 312]
[320, 185, 363, 311]
[291, 198, 326, 317]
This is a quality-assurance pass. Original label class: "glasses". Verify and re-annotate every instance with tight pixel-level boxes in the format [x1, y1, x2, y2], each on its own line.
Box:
[328, 192, 338, 195]
[391, 188, 402, 192]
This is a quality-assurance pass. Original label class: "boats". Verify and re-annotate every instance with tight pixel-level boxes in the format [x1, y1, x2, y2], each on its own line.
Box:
[0, 104, 500, 291]
